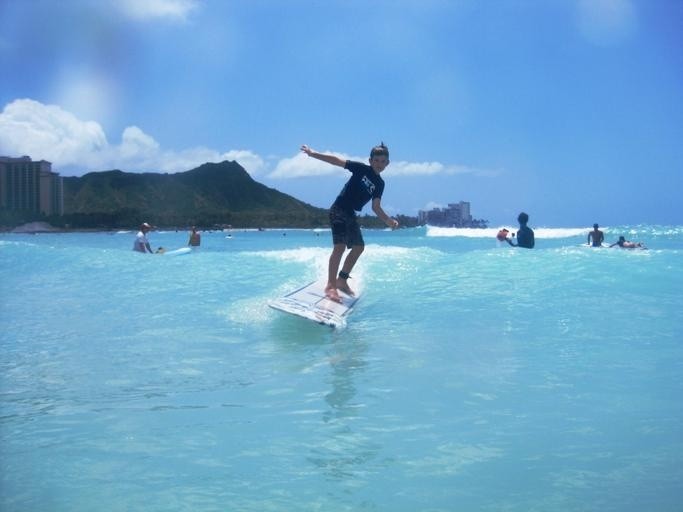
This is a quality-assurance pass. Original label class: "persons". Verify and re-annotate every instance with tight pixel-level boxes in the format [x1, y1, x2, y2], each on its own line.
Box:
[588, 223, 604, 246]
[301, 141, 399, 304]
[189, 225, 201, 247]
[132, 222, 153, 252]
[505, 211, 534, 249]
[609, 236, 643, 248]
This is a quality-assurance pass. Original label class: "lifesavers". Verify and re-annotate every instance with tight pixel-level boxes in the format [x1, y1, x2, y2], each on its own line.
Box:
[161, 247, 192, 256]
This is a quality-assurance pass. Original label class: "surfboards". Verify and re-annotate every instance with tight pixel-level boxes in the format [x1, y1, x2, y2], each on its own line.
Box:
[615, 245, 649, 250]
[269, 271, 363, 329]
[580, 241, 610, 248]
